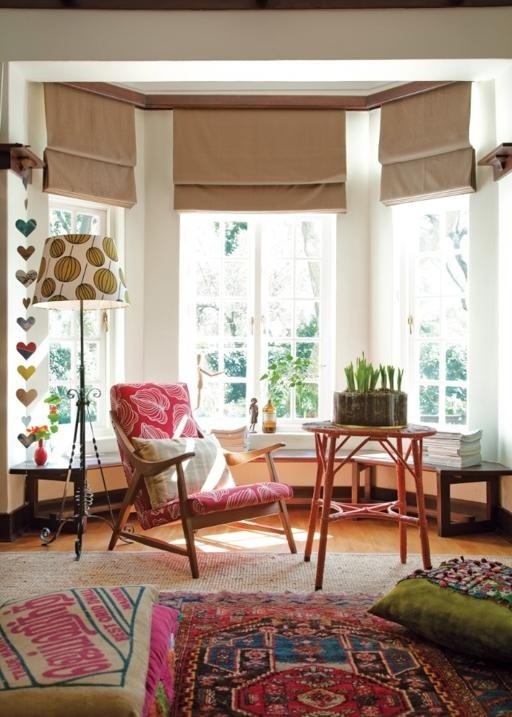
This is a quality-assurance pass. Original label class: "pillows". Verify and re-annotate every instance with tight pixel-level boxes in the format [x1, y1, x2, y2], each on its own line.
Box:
[0, 586, 182, 717]
[366, 558, 512, 668]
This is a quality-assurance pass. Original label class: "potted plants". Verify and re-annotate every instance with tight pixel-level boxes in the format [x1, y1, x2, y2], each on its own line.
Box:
[333, 354, 409, 428]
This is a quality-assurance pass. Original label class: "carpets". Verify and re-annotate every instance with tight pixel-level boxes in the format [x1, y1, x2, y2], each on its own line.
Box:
[179, 592, 512, 717]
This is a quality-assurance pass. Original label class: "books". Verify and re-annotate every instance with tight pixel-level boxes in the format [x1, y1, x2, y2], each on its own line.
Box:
[417, 424, 483, 468]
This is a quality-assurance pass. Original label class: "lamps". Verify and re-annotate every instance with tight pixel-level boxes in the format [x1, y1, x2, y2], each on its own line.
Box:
[32, 233, 126, 559]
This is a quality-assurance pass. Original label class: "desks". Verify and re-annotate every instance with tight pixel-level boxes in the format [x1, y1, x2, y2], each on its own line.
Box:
[302, 422, 435, 591]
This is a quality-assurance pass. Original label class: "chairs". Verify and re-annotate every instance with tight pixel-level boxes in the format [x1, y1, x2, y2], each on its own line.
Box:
[108, 381, 297, 579]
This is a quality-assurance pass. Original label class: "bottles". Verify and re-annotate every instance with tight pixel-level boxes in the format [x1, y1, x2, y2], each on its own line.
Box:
[33, 437, 48, 466]
[262, 399, 276, 433]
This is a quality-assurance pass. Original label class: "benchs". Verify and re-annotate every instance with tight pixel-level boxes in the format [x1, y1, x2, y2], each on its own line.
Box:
[11, 447, 512, 539]
